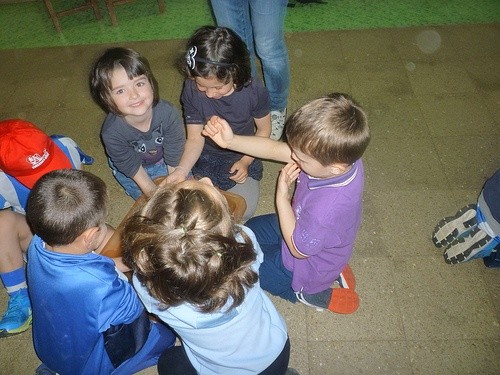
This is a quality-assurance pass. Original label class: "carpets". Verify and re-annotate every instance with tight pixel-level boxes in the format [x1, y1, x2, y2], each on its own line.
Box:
[0, 0, 500, 50]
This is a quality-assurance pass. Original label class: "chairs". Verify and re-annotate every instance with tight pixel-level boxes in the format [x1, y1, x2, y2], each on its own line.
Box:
[43, 0, 164, 33]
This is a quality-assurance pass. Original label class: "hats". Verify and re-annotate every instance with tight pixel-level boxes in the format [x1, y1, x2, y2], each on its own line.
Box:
[0, 119, 72, 190]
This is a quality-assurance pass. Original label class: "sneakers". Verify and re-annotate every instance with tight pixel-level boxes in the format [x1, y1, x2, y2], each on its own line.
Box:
[269, 108, 287, 141]
[1, 287, 32, 337]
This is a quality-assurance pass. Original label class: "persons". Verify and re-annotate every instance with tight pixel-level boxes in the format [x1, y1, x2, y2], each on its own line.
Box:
[89, 45, 187, 201]
[210, 0, 290, 142]
[26, 168, 176, 375]
[156, 26, 272, 225]
[201, 92, 372, 314]
[119, 177, 297, 375]
[431, 169, 499, 269]
[0, 118, 115, 336]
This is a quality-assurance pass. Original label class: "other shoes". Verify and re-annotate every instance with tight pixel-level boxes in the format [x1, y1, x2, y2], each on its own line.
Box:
[336, 264, 357, 289]
[296, 287, 360, 314]
[443, 227, 498, 265]
[431, 203, 479, 249]
